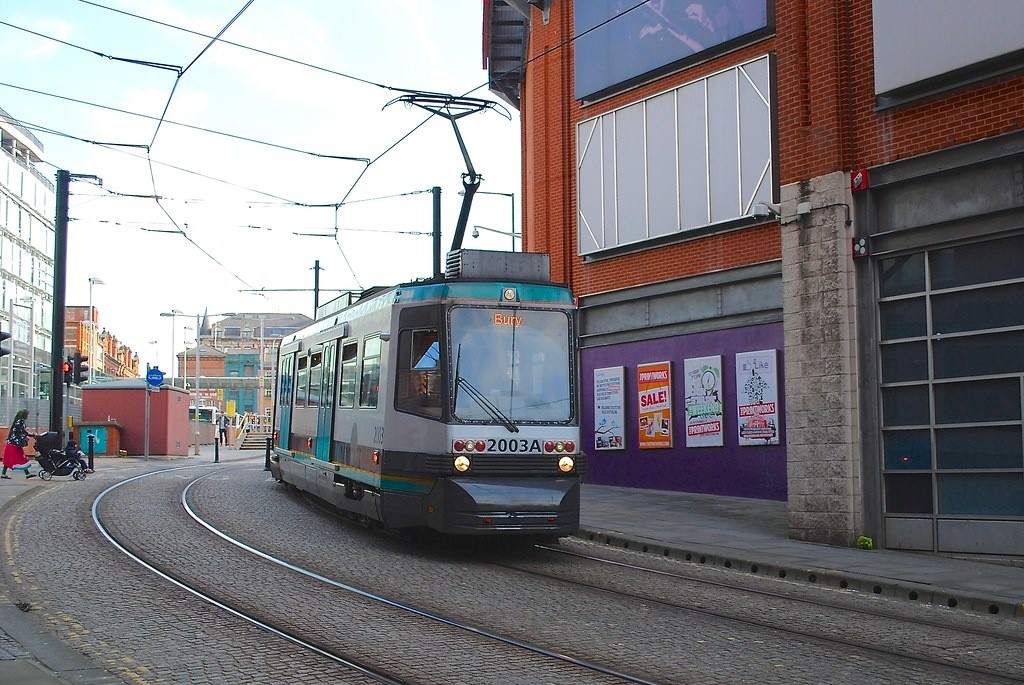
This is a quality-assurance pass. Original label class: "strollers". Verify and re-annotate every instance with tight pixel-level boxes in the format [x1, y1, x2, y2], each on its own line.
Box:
[33, 431, 86, 481]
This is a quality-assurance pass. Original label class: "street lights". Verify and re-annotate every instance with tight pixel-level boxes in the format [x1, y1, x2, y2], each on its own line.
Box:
[172, 309, 183, 386]
[159, 312, 236, 456]
[88, 277, 105, 384]
[148, 340, 157, 365]
[458, 190, 514, 252]
[231, 313, 294, 433]
[182, 326, 194, 389]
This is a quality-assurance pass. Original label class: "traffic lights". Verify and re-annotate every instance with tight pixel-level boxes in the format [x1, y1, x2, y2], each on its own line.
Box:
[63, 361, 73, 387]
[0, 331, 11, 358]
[74, 349, 88, 386]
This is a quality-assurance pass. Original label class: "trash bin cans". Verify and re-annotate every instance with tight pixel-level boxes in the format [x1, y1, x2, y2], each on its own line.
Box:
[119, 450, 127, 456]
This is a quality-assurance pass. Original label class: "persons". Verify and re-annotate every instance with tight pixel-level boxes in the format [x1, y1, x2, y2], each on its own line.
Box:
[65, 441, 93, 474]
[240, 411, 255, 432]
[1, 409, 36, 480]
[216, 410, 230, 446]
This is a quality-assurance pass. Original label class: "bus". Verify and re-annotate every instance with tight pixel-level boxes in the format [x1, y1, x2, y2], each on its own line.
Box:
[189, 405, 219, 438]
[269, 248, 588, 543]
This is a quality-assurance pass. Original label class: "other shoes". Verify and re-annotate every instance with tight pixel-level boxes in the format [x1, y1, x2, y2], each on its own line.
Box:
[84, 467, 93, 473]
[225, 444, 227, 446]
[26, 474, 37, 479]
[1, 475, 12, 479]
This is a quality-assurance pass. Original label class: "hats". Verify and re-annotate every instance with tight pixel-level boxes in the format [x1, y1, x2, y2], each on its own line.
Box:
[68, 440, 76, 446]
